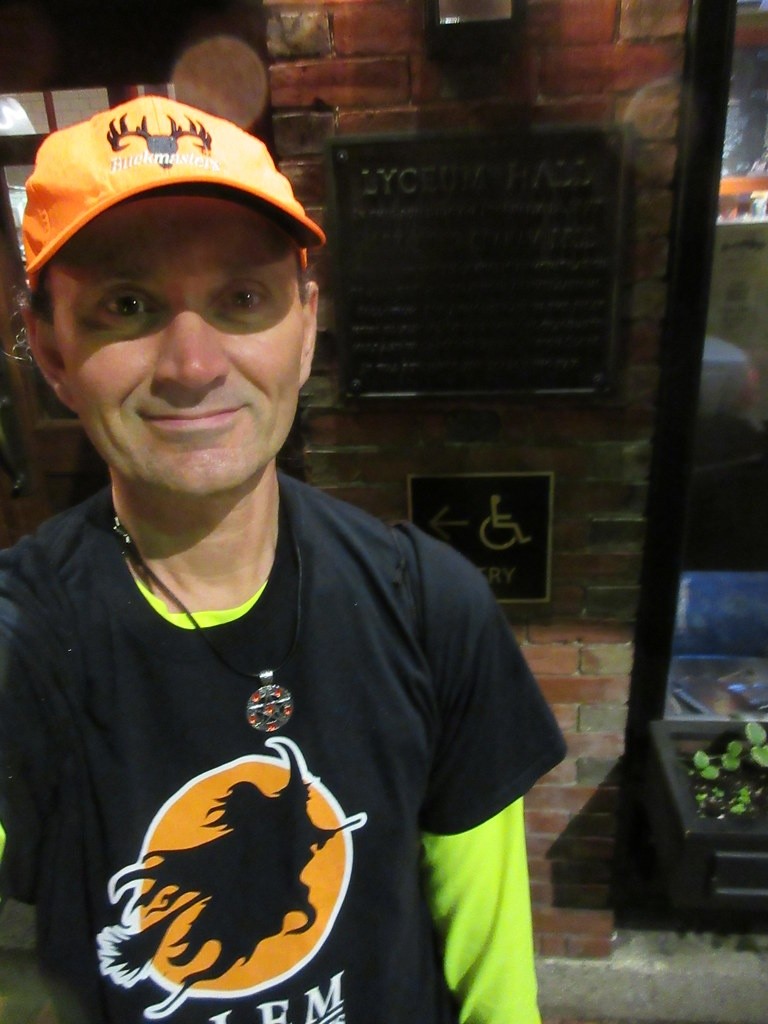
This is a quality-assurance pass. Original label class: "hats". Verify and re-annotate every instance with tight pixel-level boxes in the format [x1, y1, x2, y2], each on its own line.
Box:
[23, 94, 328, 290]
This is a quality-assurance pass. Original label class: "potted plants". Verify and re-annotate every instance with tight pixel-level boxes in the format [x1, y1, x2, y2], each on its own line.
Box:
[644, 713, 768, 914]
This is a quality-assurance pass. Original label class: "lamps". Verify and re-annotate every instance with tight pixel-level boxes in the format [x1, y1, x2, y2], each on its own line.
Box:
[425, 1, 524, 62]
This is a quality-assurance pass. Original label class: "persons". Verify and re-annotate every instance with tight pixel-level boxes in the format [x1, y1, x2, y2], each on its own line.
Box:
[0, 95, 570, 1024]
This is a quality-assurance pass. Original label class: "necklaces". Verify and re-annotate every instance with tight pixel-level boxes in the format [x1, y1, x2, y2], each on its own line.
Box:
[112, 517, 303, 734]
